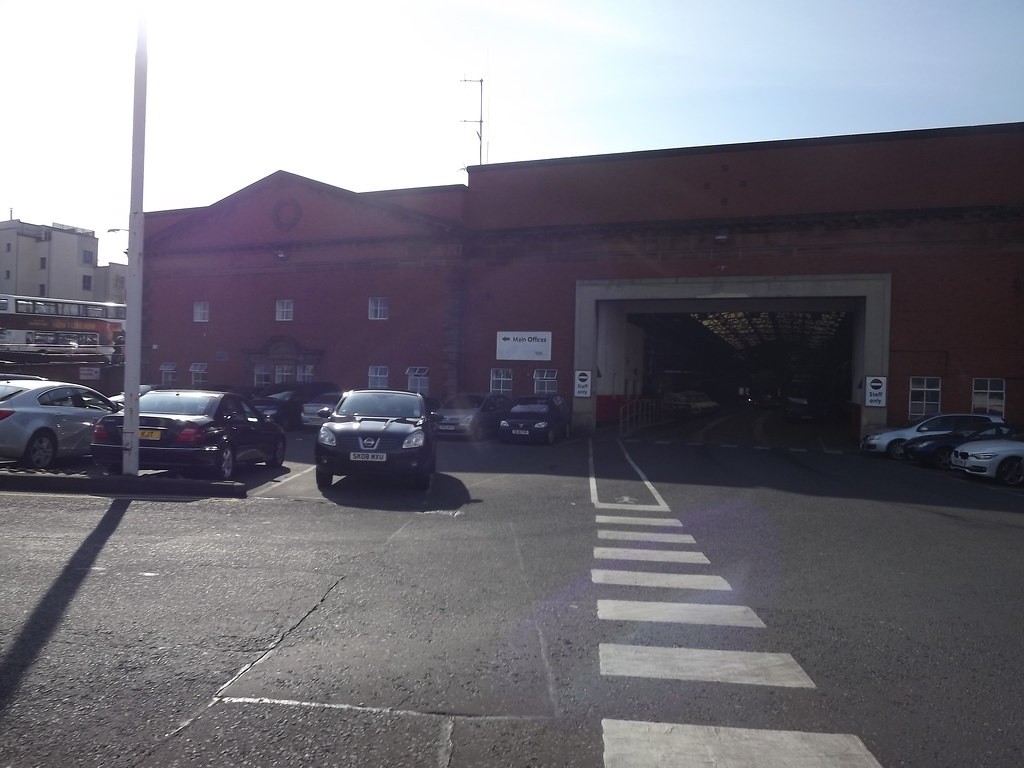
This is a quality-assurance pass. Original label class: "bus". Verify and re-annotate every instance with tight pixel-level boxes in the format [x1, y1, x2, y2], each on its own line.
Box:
[0, 293, 128, 369]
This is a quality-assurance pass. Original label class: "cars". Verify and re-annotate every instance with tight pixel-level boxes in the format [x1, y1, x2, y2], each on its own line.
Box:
[665, 382, 833, 424]
[860, 413, 1018, 464]
[0, 380, 125, 472]
[900, 424, 1021, 472]
[497, 394, 571, 445]
[98, 380, 321, 432]
[89, 389, 287, 484]
[951, 425, 1024, 489]
[314, 389, 445, 494]
[429, 393, 513, 444]
[301, 392, 342, 427]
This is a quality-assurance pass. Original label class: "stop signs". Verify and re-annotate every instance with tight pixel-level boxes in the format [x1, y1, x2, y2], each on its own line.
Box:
[870, 379, 882, 390]
[578, 372, 588, 382]
